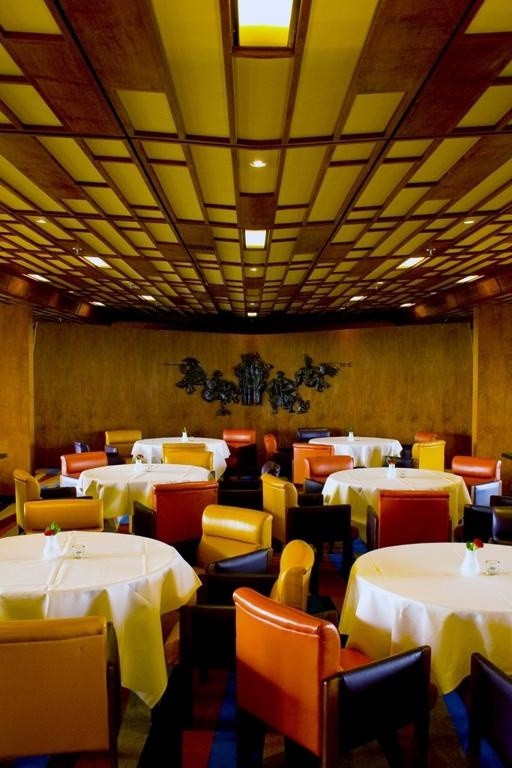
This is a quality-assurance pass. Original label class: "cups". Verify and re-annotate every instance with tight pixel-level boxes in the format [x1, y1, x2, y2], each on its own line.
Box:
[145, 464, 154, 473]
[398, 470, 406, 479]
[484, 560, 501, 575]
[72, 544, 86, 559]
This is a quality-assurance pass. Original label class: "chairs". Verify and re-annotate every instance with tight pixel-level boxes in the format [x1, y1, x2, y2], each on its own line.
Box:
[0, 615, 120, 767]
[233, 587, 437, 767]
[468, 650, 512, 768]
[164, 538, 315, 684]
